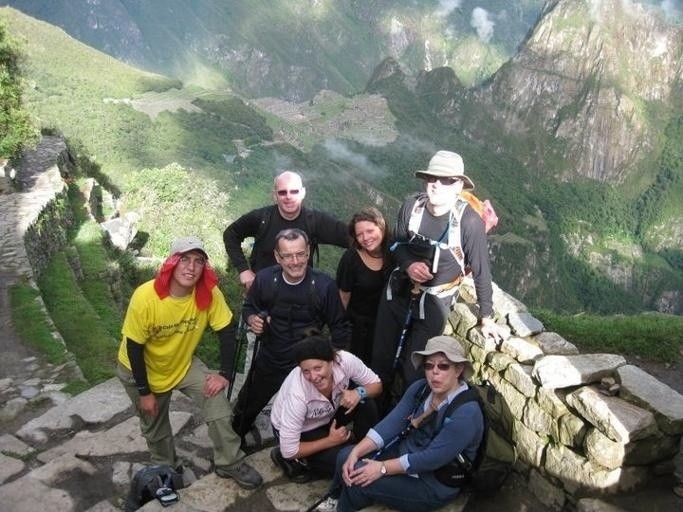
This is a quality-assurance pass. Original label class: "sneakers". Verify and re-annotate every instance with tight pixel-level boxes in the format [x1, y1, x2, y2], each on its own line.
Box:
[314, 495, 339, 512]
[268, 445, 314, 485]
[213, 459, 263, 490]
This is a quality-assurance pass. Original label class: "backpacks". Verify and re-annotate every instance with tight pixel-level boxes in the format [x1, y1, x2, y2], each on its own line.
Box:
[122, 463, 185, 512]
[407, 191, 500, 284]
[414, 378, 520, 500]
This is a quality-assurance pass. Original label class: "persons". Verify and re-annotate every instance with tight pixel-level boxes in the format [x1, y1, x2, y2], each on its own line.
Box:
[315, 335, 485, 512]
[221, 151, 496, 480]
[114, 234, 263, 492]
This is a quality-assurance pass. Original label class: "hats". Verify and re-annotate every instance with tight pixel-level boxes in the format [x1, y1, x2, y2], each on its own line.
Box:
[411, 149, 476, 193]
[409, 334, 474, 372]
[169, 234, 211, 260]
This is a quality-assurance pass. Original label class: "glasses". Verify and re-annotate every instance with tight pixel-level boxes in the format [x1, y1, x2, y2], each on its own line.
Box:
[424, 174, 463, 187]
[276, 247, 307, 261]
[274, 187, 303, 196]
[177, 254, 205, 266]
[421, 360, 457, 372]
